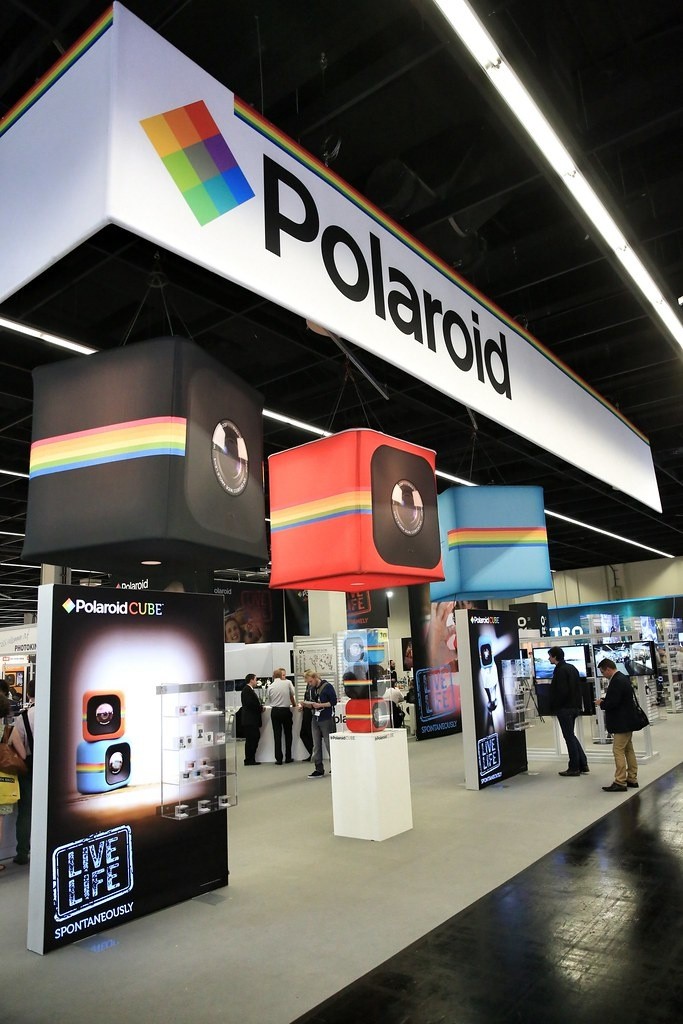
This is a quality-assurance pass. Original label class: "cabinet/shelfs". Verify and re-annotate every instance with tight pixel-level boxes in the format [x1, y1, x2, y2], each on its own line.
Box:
[154, 679, 238, 822]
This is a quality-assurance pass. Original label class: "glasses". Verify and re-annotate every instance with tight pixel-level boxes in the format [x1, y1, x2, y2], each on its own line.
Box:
[601, 671, 605, 673]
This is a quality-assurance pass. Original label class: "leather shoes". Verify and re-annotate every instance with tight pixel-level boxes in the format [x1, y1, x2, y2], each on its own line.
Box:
[626, 780, 638, 788]
[602, 783, 627, 792]
[580, 766, 589, 772]
[559, 769, 580, 776]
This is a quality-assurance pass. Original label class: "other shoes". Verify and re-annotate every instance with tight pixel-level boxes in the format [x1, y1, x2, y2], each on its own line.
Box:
[13, 855, 28, 865]
[308, 771, 322, 778]
[0, 864, 6, 870]
[275, 761, 282, 765]
[244, 760, 261, 765]
[302, 756, 312, 762]
[285, 759, 294, 763]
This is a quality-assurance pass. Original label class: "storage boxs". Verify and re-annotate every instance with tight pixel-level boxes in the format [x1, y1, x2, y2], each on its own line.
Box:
[333, 628, 398, 736]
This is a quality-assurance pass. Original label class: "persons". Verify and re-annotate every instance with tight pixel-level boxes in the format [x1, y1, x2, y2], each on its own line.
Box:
[404, 679, 416, 714]
[225, 618, 262, 643]
[384, 660, 397, 684]
[428, 600, 488, 667]
[383, 682, 405, 717]
[595, 659, 643, 791]
[241, 674, 266, 766]
[0, 679, 35, 871]
[297, 669, 337, 778]
[267, 668, 297, 765]
[547, 647, 589, 776]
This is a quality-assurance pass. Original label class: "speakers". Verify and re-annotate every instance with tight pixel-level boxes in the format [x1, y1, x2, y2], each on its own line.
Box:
[75, 689, 131, 794]
[21, 336, 553, 603]
[478, 635, 495, 668]
[343, 630, 390, 733]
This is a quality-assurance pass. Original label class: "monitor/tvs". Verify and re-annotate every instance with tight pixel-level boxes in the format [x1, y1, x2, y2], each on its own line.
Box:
[593, 641, 657, 678]
[532, 645, 592, 678]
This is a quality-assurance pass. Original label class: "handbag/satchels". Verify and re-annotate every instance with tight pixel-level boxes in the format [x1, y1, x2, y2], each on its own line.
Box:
[0, 724, 27, 774]
[329, 716, 337, 733]
[634, 705, 649, 731]
